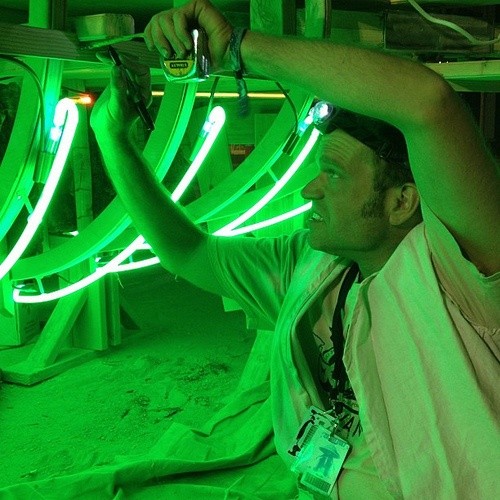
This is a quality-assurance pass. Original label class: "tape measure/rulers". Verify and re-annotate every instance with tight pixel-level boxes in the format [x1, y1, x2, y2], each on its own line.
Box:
[83, 27, 208, 83]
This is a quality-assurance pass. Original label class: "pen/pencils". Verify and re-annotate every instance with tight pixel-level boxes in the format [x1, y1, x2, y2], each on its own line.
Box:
[108, 45, 155, 131]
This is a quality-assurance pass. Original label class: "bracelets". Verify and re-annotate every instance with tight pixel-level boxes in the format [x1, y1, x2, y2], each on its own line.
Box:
[228, 25, 252, 115]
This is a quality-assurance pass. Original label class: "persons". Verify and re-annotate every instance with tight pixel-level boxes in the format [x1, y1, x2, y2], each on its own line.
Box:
[90, 0, 499, 500]
